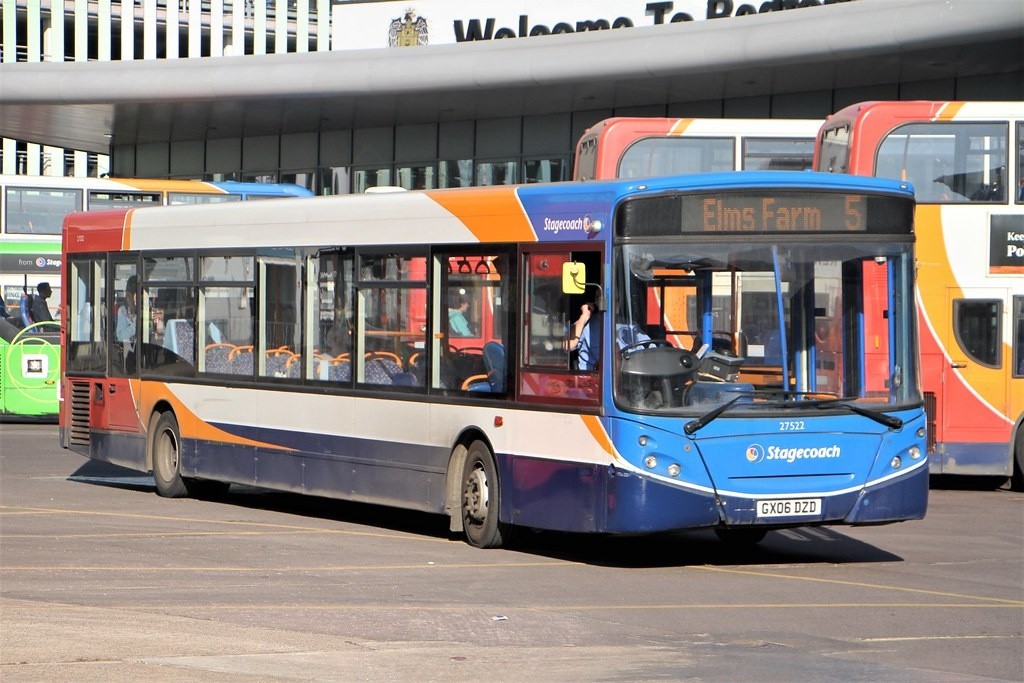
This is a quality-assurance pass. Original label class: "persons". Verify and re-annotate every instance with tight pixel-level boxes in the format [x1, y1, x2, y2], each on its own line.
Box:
[561, 284, 657, 371]
[31, 281, 57, 330]
[116, 276, 164, 370]
[444, 294, 478, 338]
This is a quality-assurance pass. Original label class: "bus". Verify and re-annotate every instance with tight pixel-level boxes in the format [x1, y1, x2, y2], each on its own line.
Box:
[575, 117, 989, 404]
[60, 168, 929, 550]
[1, 173, 316, 425]
[811, 98, 1023, 490]
[405, 254, 569, 365]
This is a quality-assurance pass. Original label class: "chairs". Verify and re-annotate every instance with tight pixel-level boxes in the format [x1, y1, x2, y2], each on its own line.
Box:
[461, 375, 491, 392]
[20, 294, 37, 333]
[364, 352, 404, 384]
[229, 345, 254, 376]
[208, 317, 250, 352]
[450, 347, 484, 382]
[401, 341, 427, 367]
[482, 341, 505, 392]
[286, 353, 324, 380]
[265, 349, 297, 377]
[316, 358, 351, 382]
[163, 319, 213, 366]
[206, 343, 241, 374]
[407, 351, 454, 390]
